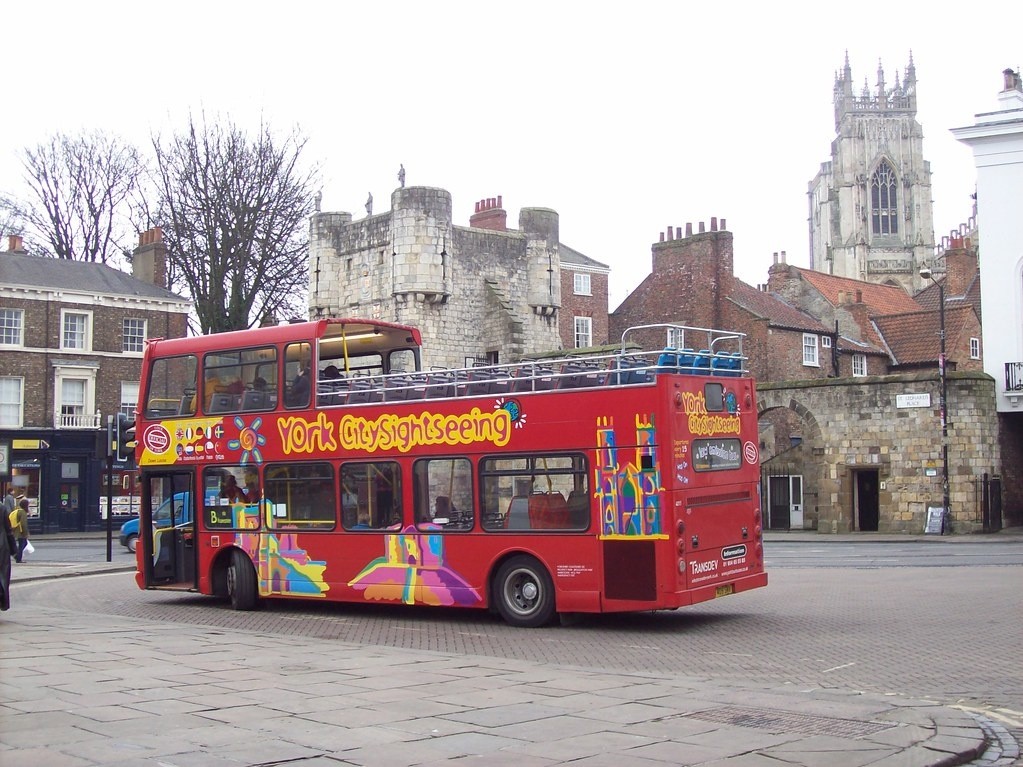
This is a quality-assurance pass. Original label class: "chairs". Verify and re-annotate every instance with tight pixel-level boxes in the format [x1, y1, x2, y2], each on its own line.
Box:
[176, 346, 746, 416]
[442, 487, 589, 531]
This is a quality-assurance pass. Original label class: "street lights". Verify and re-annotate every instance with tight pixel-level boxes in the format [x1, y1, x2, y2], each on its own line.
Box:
[918, 269, 952, 538]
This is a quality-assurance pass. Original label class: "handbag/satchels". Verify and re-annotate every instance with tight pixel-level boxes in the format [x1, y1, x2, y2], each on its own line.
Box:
[23, 538, 34, 553]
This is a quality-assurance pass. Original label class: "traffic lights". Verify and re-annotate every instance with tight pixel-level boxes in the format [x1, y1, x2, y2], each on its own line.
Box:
[134, 317, 768, 629]
[116, 413, 137, 463]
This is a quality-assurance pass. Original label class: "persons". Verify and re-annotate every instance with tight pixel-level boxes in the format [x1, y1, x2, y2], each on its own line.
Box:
[398, 164, 405, 187]
[190, 365, 350, 414]
[0, 488, 30, 611]
[365, 192, 373, 217]
[215, 471, 458, 529]
[315, 191, 322, 212]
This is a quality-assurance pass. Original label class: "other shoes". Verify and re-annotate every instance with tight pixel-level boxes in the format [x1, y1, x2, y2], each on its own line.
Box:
[17, 560, 26, 563]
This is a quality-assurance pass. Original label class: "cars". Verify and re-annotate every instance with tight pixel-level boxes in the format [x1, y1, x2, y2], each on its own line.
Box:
[118, 490, 220, 553]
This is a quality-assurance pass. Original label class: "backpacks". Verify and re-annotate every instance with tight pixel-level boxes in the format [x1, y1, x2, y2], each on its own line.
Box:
[9, 509, 23, 528]
